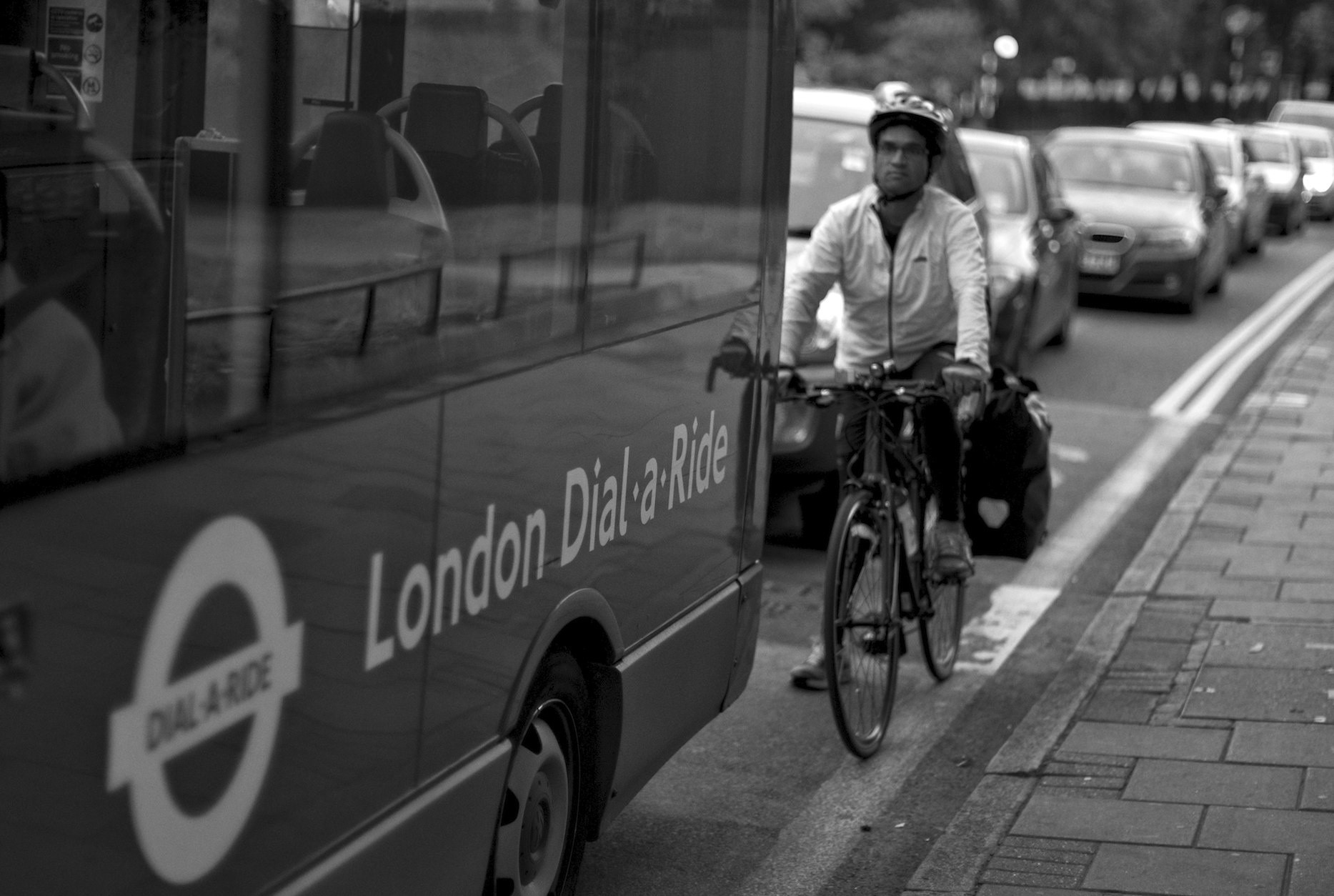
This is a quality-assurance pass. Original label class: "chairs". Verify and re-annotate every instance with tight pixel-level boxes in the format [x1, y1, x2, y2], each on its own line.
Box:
[155, 83, 668, 417]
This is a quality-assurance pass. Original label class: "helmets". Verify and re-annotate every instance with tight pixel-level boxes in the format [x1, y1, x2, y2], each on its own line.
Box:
[867, 93, 948, 147]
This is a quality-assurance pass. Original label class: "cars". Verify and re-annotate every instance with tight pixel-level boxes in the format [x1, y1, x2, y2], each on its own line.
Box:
[774, 79, 1333, 551]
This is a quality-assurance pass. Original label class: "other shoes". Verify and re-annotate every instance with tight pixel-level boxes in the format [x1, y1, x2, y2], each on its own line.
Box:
[788, 642, 849, 691]
[933, 522, 975, 580]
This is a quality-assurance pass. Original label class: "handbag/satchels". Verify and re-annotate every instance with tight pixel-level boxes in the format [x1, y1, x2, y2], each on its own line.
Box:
[963, 362, 1053, 561]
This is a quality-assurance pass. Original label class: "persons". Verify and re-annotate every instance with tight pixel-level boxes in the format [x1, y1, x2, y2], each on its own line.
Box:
[0, 169, 124, 482]
[721, 280, 761, 546]
[777, 96, 992, 688]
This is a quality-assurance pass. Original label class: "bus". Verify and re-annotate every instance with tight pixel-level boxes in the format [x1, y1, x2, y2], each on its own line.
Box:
[1, 0, 798, 896]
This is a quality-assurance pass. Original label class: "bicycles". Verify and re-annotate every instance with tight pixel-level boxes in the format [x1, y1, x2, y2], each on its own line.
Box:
[775, 358, 983, 759]
